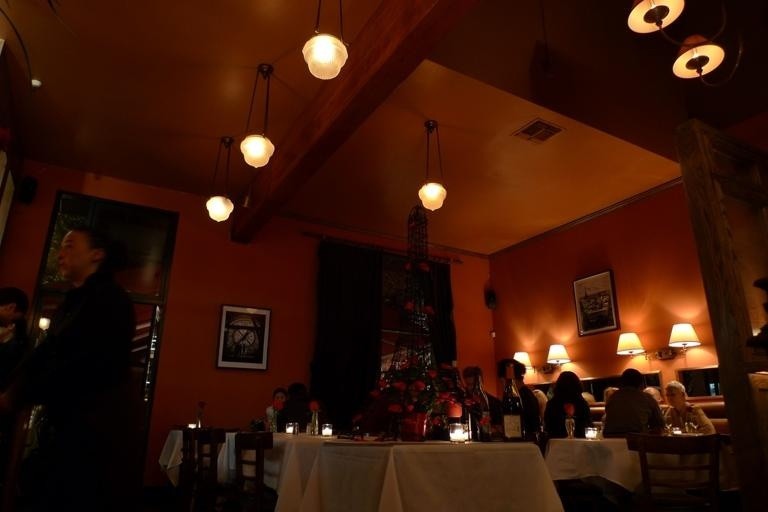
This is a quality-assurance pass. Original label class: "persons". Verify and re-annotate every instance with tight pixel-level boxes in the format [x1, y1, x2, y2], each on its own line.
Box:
[267, 382, 321, 430]
[602, 368, 663, 437]
[1, 226, 145, 511]
[1, 289, 39, 486]
[462, 356, 548, 443]
[664, 381, 716, 435]
[546, 371, 601, 438]
[581, 384, 662, 410]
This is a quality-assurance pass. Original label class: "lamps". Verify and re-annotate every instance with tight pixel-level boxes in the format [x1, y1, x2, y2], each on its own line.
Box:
[418, 121, 448, 211]
[302, 0, 351, 80]
[240, 63, 276, 168]
[206, 136, 234, 222]
[514, 343, 570, 375]
[627, 0, 727, 48]
[672, 17, 743, 88]
[617, 323, 702, 360]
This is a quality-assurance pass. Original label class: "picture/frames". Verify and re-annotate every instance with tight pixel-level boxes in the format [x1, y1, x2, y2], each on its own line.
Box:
[572, 269, 621, 337]
[215, 303, 272, 371]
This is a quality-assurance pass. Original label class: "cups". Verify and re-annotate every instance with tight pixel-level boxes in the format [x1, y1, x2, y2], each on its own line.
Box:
[584, 427, 598, 438]
[285, 422, 294, 433]
[322, 423, 332, 437]
[189, 423, 196, 429]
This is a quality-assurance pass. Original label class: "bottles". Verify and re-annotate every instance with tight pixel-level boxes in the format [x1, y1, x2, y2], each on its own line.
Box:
[447, 360, 524, 441]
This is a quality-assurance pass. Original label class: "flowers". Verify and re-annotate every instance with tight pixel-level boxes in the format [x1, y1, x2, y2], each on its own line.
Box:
[369, 363, 476, 425]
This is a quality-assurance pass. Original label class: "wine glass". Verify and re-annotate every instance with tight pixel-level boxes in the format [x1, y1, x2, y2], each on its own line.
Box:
[664, 416, 673, 435]
